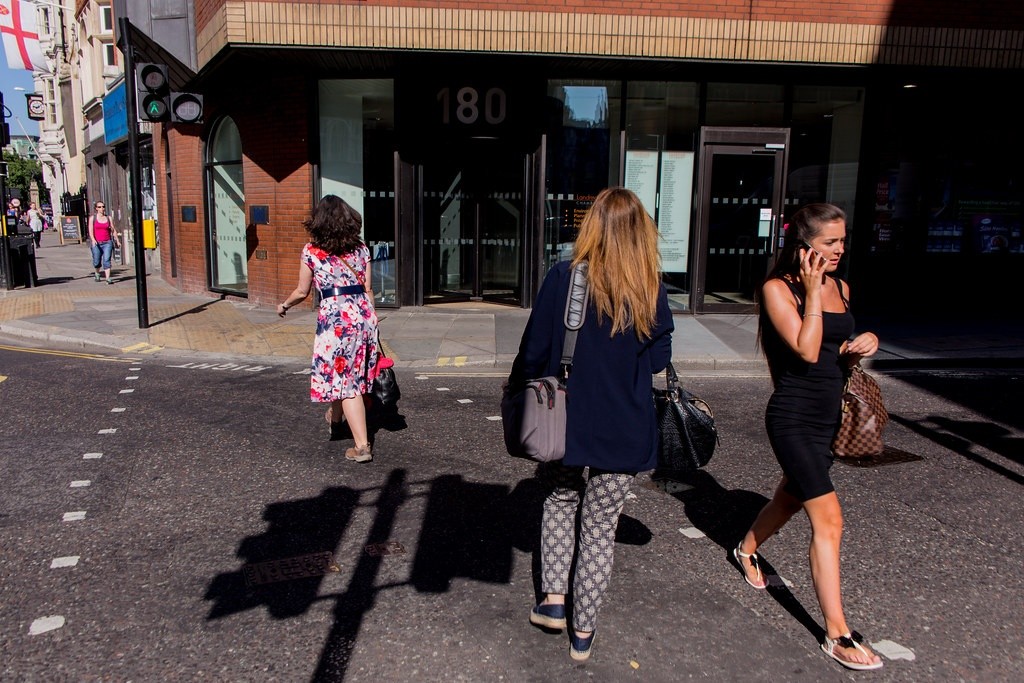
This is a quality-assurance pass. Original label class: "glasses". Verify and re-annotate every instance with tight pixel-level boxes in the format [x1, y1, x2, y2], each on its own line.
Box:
[98, 206, 105, 208]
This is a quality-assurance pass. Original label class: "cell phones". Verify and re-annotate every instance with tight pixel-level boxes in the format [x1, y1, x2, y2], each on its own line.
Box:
[793, 239, 827, 270]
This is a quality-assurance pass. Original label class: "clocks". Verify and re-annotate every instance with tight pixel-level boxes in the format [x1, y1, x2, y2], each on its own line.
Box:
[26, 94, 45, 118]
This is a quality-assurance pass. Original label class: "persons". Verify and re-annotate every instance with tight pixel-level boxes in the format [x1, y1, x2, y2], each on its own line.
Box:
[26, 202, 43, 249]
[276, 194, 379, 462]
[7, 202, 26, 226]
[88, 201, 121, 284]
[506, 187, 675, 662]
[732, 201, 886, 670]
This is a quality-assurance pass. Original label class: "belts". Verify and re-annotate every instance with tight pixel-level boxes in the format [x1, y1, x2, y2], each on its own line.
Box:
[319, 285, 366, 302]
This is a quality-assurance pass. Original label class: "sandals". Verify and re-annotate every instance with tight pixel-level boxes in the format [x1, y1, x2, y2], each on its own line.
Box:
[734, 540, 769, 589]
[821, 631, 883, 670]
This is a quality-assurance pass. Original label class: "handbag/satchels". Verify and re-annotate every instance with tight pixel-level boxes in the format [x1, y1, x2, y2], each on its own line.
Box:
[652, 362, 718, 472]
[502, 376, 567, 462]
[834, 352, 888, 457]
[373, 337, 401, 407]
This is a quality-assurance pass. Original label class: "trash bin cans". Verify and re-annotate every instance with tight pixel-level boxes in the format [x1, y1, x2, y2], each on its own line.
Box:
[7, 226, 39, 288]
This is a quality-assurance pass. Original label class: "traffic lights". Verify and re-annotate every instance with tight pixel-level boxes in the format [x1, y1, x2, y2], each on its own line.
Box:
[136, 61, 168, 120]
[168, 92, 205, 123]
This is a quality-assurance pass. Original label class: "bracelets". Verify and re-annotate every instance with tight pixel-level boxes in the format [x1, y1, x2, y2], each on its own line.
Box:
[803, 312, 823, 319]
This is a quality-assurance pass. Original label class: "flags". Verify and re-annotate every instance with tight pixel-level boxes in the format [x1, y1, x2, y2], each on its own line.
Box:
[0, 0, 53, 75]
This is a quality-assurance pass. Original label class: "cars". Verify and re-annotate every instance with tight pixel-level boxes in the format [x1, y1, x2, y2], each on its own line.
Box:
[42, 205, 53, 215]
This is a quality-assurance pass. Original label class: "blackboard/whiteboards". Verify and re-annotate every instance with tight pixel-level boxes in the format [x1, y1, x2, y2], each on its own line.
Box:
[60, 216, 81, 241]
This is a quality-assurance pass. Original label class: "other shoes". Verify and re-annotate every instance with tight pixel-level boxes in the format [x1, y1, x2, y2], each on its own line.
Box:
[94, 274, 100, 282]
[111, 235, 117, 248]
[326, 407, 343, 435]
[570, 627, 596, 660]
[531, 600, 567, 629]
[105, 279, 112, 284]
[345, 443, 373, 462]
